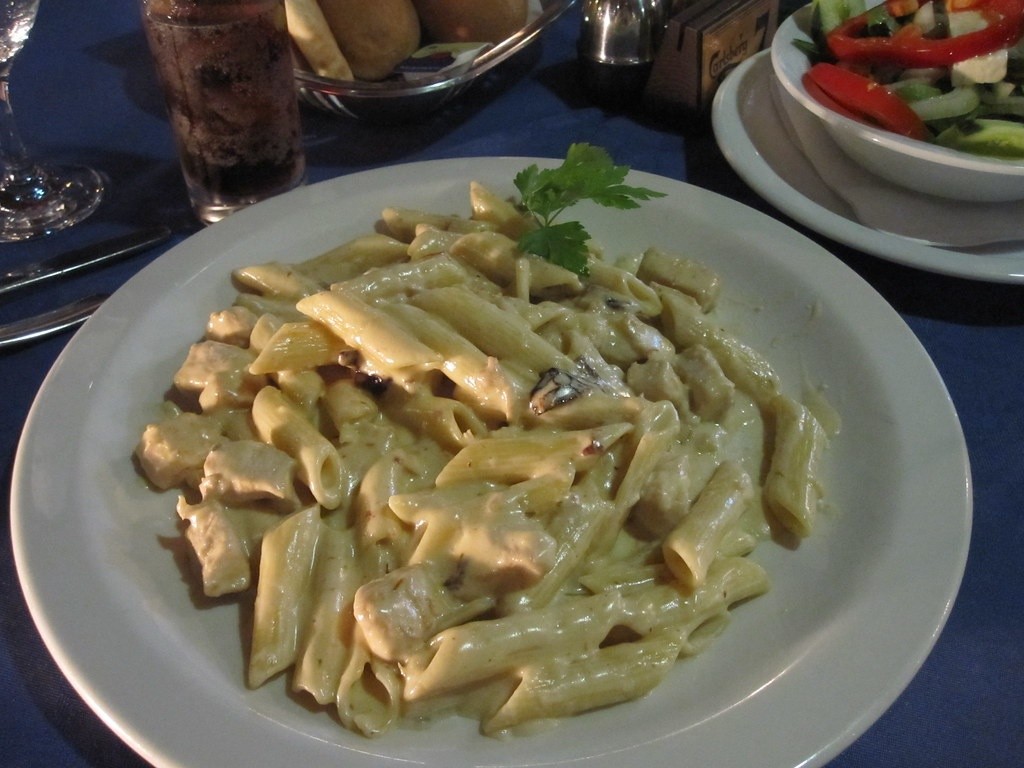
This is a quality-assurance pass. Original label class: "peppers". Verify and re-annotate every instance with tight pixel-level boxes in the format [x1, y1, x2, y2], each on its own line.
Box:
[806, 62, 928, 139]
[826, 0, 1024, 67]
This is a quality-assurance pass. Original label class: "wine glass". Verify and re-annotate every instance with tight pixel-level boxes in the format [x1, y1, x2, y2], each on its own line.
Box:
[0, 0, 105, 244]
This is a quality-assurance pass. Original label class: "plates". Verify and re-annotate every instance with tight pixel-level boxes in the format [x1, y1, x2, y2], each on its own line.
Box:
[6, 158, 973, 768]
[292, 1, 581, 127]
[710, 45, 1024, 289]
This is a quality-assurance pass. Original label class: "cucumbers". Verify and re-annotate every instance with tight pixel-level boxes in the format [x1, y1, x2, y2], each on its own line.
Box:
[801, 0, 1024, 164]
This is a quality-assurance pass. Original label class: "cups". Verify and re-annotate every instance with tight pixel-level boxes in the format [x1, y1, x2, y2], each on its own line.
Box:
[138, 0, 309, 230]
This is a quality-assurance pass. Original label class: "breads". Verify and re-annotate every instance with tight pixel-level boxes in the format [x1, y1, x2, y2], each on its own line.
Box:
[281, 0, 530, 85]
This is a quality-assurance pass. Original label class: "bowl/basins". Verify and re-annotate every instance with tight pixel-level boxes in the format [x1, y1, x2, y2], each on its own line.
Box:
[770, 0, 1024, 207]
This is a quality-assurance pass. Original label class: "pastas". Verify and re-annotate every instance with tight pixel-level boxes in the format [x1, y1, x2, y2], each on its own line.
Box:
[135, 179, 830, 742]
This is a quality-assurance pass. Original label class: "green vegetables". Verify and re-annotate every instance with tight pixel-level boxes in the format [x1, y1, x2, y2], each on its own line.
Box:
[514, 140, 670, 280]
